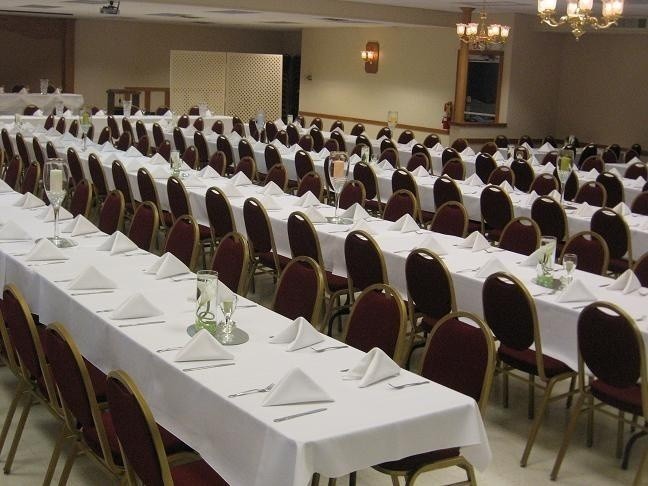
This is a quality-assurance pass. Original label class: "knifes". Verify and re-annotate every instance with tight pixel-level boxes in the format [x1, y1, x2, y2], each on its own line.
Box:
[119, 321, 165, 327]
[273, 408, 328, 422]
[183, 363, 236, 372]
[71, 290, 113, 296]
[28, 261, 64, 265]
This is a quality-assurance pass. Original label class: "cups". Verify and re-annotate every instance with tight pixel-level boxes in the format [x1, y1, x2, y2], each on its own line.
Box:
[55, 100, 65, 117]
[123, 100, 132, 118]
[198, 102, 208, 117]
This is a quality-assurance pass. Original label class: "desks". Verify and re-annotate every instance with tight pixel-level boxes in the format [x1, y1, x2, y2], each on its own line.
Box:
[1, 90, 85, 115]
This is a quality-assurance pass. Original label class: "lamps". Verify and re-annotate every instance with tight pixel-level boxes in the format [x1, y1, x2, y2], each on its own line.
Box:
[360, 48, 375, 65]
[536, 0, 625, 42]
[455, 1, 512, 56]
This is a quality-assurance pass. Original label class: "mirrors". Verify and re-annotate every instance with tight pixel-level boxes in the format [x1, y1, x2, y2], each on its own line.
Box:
[456, 47, 503, 124]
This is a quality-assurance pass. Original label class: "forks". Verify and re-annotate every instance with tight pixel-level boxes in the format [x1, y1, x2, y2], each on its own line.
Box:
[310, 345, 349, 353]
[228, 383, 276, 398]
[387, 382, 430, 390]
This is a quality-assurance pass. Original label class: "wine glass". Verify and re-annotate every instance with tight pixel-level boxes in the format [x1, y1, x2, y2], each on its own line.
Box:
[79, 110, 92, 152]
[254, 111, 266, 145]
[36, 158, 78, 249]
[329, 150, 349, 224]
[219, 294, 236, 344]
[388, 110, 399, 141]
[556, 149, 574, 206]
[563, 254, 578, 285]
